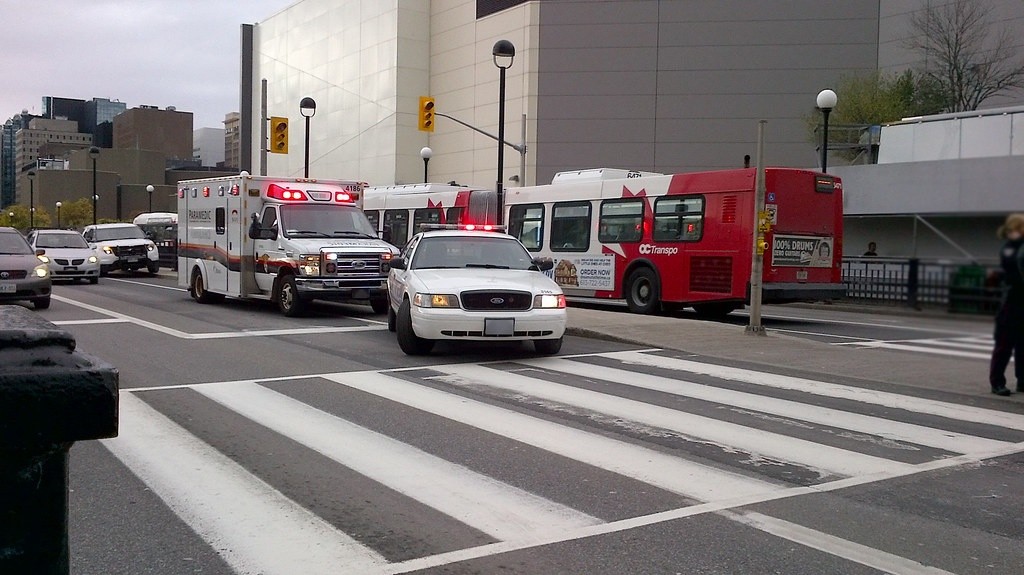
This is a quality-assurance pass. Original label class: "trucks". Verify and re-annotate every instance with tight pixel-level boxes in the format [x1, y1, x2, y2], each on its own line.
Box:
[177, 170, 401, 319]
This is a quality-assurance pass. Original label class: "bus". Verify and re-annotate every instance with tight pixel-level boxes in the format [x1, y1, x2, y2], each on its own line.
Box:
[351, 168, 848, 318]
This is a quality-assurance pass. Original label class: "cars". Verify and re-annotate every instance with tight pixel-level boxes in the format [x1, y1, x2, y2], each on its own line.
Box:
[0, 225, 52, 310]
[25, 229, 102, 285]
[386, 222, 568, 356]
[82, 222, 160, 277]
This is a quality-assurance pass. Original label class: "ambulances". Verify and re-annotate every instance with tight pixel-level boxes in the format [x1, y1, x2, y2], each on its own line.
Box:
[270, 116, 288, 154]
[418, 96, 435, 134]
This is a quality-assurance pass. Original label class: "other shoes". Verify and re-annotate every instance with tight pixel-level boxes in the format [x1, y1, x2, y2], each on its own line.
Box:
[1017, 385, 1024, 392]
[992, 385, 1010, 396]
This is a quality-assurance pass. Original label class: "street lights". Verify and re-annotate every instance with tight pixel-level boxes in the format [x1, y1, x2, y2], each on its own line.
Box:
[10, 212, 15, 227]
[146, 184, 154, 213]
[421, 147, 433, 183]
[89, 146, 100, 225]
[56, 201, 62, 229]
[91, 194, 99, 225]
[492, 39, 516, 225]
[815, 89, 837, 174]
[300, 96, 316, 178]
[27, 170, 36, 229]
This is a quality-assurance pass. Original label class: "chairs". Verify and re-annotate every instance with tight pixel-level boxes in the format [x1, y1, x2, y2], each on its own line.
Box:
[424, 244, 449, 266]
[482, 244, 503, 265]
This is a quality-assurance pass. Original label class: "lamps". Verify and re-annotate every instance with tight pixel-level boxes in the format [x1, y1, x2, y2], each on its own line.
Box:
[508, 175, 518, 181]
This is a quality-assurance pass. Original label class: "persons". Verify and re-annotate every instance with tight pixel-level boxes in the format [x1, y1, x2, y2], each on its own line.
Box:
[600, 221, 613, 241]
[988, 212, 1024, 396]
[812, 242, 831, 267]
[863, 242, 876, 256]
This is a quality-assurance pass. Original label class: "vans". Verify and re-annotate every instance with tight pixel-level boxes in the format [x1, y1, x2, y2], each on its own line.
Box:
[133, 212, 178, 272]
